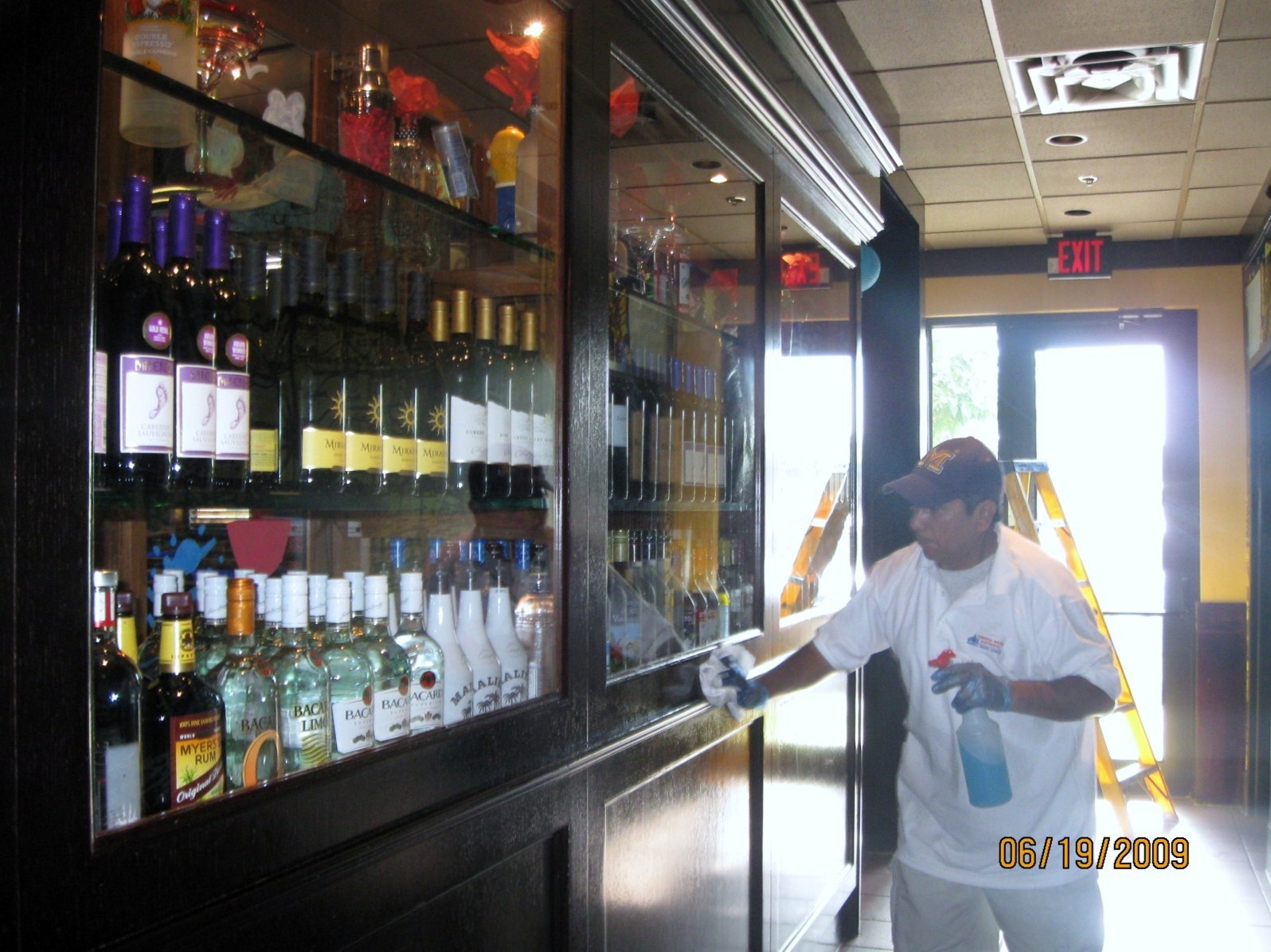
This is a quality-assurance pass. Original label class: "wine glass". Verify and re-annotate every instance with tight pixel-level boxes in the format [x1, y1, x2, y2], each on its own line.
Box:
[193, 0, 265, 176]
[617, 224, 661, 295]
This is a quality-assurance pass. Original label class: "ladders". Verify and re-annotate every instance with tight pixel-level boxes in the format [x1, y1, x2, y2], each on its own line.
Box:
[1001, 457, 1189, 833]
[777, 461, 853, 618]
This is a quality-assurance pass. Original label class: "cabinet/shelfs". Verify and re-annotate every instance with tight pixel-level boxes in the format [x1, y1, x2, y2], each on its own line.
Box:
[0, 0, 905, 952]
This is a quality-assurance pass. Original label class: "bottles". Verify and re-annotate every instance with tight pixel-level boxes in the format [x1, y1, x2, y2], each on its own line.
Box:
[956, 680, 1012, 808]
[609, 341, 734, 501]
[607, 530, 754, 674]
[339, 48, 395, 177]
[93, 540, 555, 832]
[666, 224, 692, 313]
[95, 171, 554, 500]
[431, 120, 470, 209]
[120, 0, 197, 147]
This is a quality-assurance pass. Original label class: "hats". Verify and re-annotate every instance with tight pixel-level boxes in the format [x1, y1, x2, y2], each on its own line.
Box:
[884, 436, 1001, 507]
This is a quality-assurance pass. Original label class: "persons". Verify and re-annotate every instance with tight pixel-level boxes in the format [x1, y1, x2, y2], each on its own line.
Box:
[424, 443, 684, 662]
[713, 435, 1122, 952]
